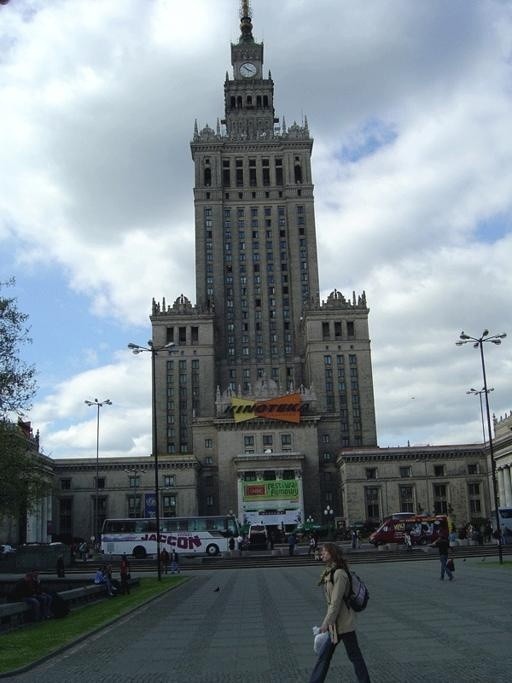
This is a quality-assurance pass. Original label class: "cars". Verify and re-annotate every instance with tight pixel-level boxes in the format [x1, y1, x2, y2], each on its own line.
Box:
[492, 507, 512, 536]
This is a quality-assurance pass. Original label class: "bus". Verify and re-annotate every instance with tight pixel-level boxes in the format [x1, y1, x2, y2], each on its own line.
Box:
[248, 523, 268, 544]
[99, 515, 244, 558]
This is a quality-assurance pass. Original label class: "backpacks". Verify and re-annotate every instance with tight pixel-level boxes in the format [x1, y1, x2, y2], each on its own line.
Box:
[329, 565, 369, 612]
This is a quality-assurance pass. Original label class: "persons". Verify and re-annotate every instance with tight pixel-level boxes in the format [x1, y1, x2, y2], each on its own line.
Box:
[447, 528, 459, 547]
[94, 552, 131, 596]
[243, 536, 249, 550]
[227, 535, 234, 554]
[24, 567, 56, 619]
[428, 532, 455, 582]
[56, 554, 65, 578]
[169, 547, 181, 574]
[307, 533, 317, 554]
[404, 530, 415, 553]
[287, 532, 296, 554]
[236, 532, 243, 555]
[306, 541, 373, 681]
[492, 525, 512, 545]
[14, 575, 41, 622]
[159, 547, 169, 574]
[267, 530, 275, 549]
[68, 541, 100, 565]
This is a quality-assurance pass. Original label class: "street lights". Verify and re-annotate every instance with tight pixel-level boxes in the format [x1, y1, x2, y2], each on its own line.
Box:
[324, 505, 334, 541]
[307, 516, 314, 530]
[128, 338, 179, 581]
[123, 468, 147, 518]
[227, 510, 236, 518]
[456, 329, 507, 565]
[84, 398, 112, 553]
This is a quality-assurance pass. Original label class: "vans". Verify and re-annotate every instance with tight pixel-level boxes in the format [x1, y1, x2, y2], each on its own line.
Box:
[368, 512, 450, 547]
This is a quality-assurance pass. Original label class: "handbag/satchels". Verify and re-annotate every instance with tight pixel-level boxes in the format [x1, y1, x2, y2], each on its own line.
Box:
[446, 558, 455, 571]
